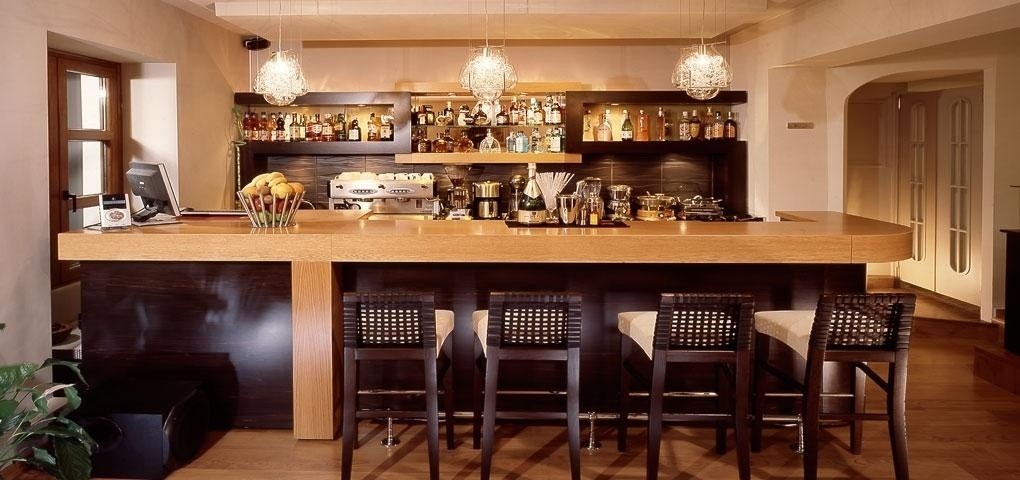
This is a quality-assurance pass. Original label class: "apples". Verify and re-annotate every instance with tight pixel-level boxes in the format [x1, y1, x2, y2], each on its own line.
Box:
[242, 183, 304, 224]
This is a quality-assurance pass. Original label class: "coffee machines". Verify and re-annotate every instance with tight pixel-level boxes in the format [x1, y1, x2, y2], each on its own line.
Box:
[327, 180, 440, 215]
[607, 185, 632, 221]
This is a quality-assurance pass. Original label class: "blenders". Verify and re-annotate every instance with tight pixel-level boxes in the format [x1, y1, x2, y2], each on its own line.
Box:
[445, 167, 471, 209]
[471, 179, 503, 219]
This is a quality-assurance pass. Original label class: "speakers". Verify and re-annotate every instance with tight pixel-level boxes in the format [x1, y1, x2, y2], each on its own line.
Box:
[98, 192, 132, 233]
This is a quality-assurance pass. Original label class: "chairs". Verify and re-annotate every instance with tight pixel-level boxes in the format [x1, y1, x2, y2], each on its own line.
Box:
[471, 288, 584, 479]
[341, 288, 454, 479]
[750, 291, 917, 479]
[614, 290, 756, 480]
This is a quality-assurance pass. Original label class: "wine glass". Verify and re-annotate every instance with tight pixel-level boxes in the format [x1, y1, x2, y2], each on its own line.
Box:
[543, 195, 556, 224]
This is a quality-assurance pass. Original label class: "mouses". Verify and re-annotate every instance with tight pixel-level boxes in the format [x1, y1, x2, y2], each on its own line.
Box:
[181, 208, 194, 213]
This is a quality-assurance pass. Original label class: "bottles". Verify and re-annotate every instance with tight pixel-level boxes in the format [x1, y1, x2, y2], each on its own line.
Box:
[415, 94, 563, 125]
[582, 178, 605, 223]
[519, 162, 547, 225]
[657, 104, 738, 142]
[638, 110, 648, 140]
[582, 109, 613, 141]
[242, 110, 393, 141]
[620, 110, 635, 140]
[508, 175, 525, 219]
[414, 127, 566, 153]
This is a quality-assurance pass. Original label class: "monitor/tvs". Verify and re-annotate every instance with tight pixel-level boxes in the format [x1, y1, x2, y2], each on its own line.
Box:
[126, 161, 183, 227]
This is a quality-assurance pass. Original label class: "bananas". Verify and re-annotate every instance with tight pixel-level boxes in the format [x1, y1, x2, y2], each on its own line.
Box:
[252, 172, 288, 188]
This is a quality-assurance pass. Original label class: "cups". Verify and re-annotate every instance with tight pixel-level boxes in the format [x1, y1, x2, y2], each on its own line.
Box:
[556, 195, 577, 224]
[336, 172, 433, 181]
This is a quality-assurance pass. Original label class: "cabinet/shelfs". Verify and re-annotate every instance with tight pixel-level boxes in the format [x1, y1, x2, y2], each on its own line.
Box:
[392, 92, 584, 165]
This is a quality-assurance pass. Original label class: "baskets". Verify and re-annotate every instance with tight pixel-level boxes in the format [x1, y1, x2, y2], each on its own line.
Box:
[236, 190, 306, 228]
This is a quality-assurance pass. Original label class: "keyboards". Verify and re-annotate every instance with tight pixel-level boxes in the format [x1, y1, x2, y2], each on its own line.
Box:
[181, 211, 254, 217]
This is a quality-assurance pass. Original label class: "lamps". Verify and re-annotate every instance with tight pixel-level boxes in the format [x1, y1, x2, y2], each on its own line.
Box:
[253, 0, 308, 107]
[670, 0, 732, 102]
[458, 0, 517, 104]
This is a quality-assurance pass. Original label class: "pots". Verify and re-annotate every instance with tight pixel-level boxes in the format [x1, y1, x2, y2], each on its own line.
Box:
[637, 195, 676, 221]
